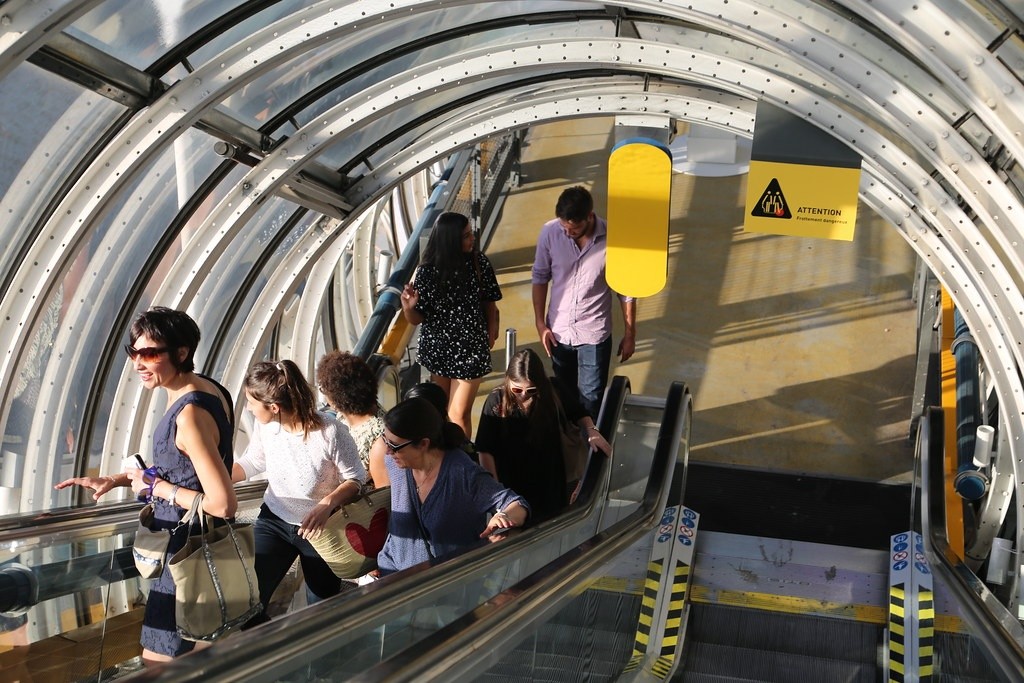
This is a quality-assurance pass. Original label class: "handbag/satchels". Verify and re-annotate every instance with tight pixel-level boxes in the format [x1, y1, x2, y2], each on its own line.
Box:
[302, 478, 391, 579]
[132, 505, 170, 579]
[168, 493, 264, 644]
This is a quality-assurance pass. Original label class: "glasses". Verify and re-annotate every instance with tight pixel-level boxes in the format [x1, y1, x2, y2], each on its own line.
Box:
[509, 380, 538, 394]
[381, 433, 412, 453]
[124, 345, 170, 363]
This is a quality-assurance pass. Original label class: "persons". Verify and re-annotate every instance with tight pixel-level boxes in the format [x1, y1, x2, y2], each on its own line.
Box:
[319, 348, 529, 575]
[401, 212, 503, 441]
[231, 359, 366, 616]
[531, 185, 637, 422]
[476, 347, 612, 522]
[54, 307, 238, 664]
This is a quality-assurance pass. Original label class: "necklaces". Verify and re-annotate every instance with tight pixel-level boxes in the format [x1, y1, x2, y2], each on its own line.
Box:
[414, 467, 435, 494]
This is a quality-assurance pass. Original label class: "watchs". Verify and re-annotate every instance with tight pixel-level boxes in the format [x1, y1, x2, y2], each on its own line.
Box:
[588, 425, 599, 432]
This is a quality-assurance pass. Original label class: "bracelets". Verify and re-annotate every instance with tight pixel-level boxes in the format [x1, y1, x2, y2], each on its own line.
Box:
[169, 485, 179, 507]
[106, 476, 115, 483]
